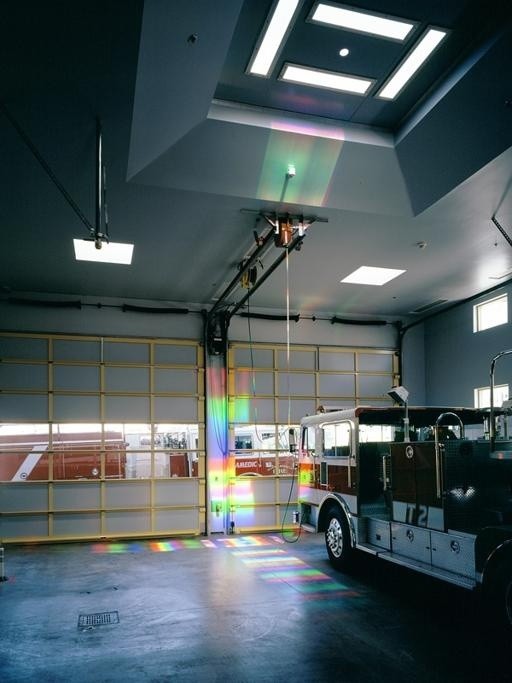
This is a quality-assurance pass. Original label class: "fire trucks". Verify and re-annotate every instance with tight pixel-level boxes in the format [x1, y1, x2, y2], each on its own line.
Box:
[291, 405, 512, 631]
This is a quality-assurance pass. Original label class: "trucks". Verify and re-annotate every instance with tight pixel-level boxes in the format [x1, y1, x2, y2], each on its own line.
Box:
[1, 423, 299, 479]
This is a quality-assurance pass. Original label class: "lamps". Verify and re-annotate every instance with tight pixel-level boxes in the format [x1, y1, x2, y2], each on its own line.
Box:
[387, 386, 411, 442]
